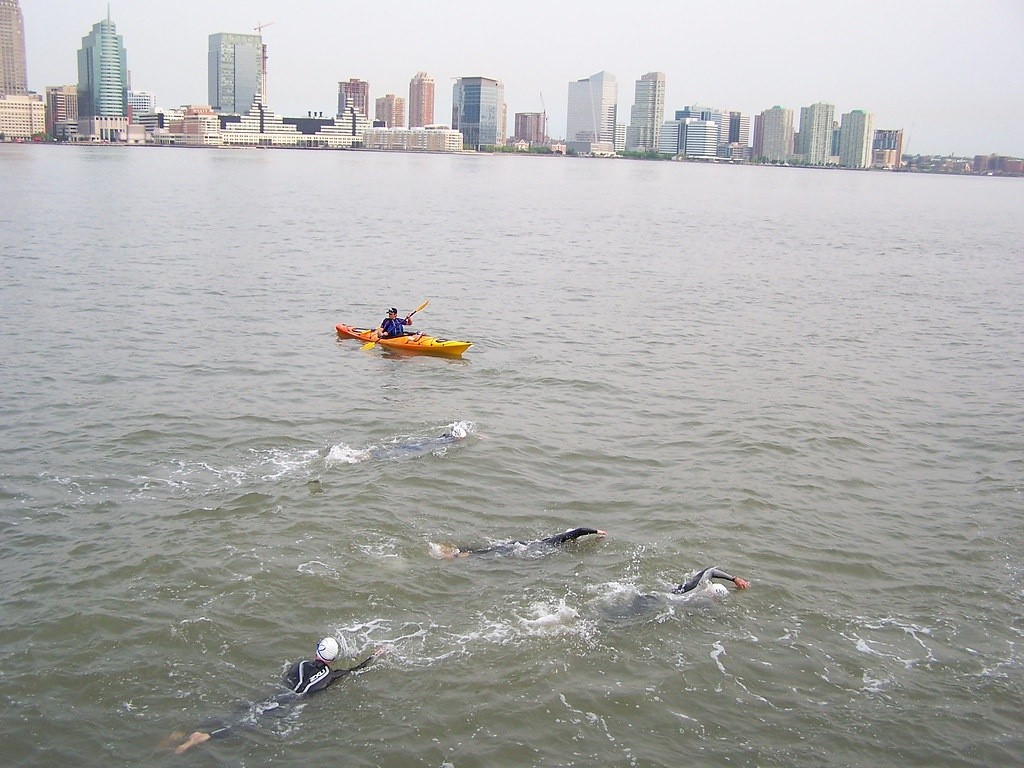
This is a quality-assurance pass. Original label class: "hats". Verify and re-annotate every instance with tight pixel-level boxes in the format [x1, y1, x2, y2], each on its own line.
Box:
[710, 583, 728, 598]
[386, 308, 397, 314]
[316, 636, 339, 662]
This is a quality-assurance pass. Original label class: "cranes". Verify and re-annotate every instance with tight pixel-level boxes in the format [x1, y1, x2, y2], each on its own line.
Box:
[253, 20, 274, 35]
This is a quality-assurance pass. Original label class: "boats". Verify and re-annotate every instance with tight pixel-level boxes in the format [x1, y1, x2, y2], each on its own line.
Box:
[335, 322, 473, 359]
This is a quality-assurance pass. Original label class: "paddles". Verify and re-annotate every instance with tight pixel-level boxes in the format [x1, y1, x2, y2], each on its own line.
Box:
[362, 299, 431, 350]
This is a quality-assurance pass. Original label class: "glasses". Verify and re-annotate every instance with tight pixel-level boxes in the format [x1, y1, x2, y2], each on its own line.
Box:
[389, 313, 395, 315]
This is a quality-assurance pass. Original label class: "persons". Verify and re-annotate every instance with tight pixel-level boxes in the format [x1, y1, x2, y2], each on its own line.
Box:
[170, 638, 371, 754]
[378, 308, 412, 338]
[561, 527, 606, 542]
[681, 567, 747, 595]
